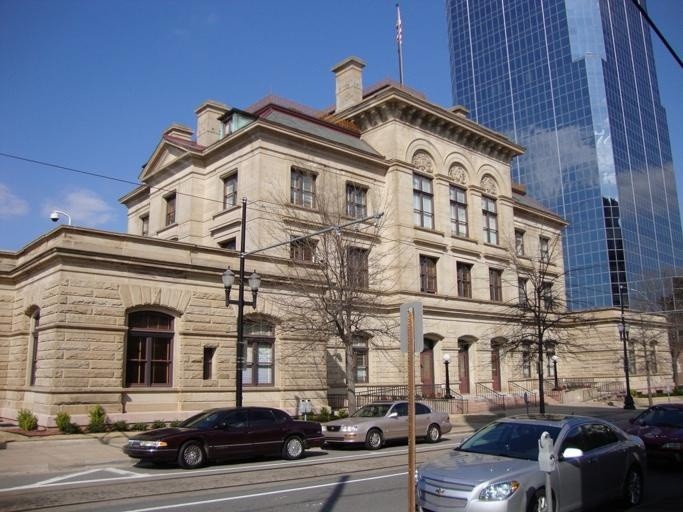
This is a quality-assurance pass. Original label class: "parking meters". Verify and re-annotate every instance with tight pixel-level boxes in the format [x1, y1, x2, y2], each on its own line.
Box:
[300, 399, 312, 422]
[537, 430, 560, 511]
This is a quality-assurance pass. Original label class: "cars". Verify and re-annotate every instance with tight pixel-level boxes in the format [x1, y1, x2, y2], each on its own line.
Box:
[321, 399, 453, 450]
[415, 412, 648, 512]
[121, 406, 327, 470]
[622, 403, 683, 472]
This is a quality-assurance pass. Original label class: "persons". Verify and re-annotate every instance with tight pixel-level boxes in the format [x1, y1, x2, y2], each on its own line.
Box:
[306, 400, 314, 415]
[300, 399, 306, 416]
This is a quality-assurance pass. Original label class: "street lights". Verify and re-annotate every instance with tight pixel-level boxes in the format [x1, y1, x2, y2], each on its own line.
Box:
[220, 196, 385, 407]
[618, 285, 635, 410]
[441, 353, 455, 399]
[551, 355, 562, 391]
